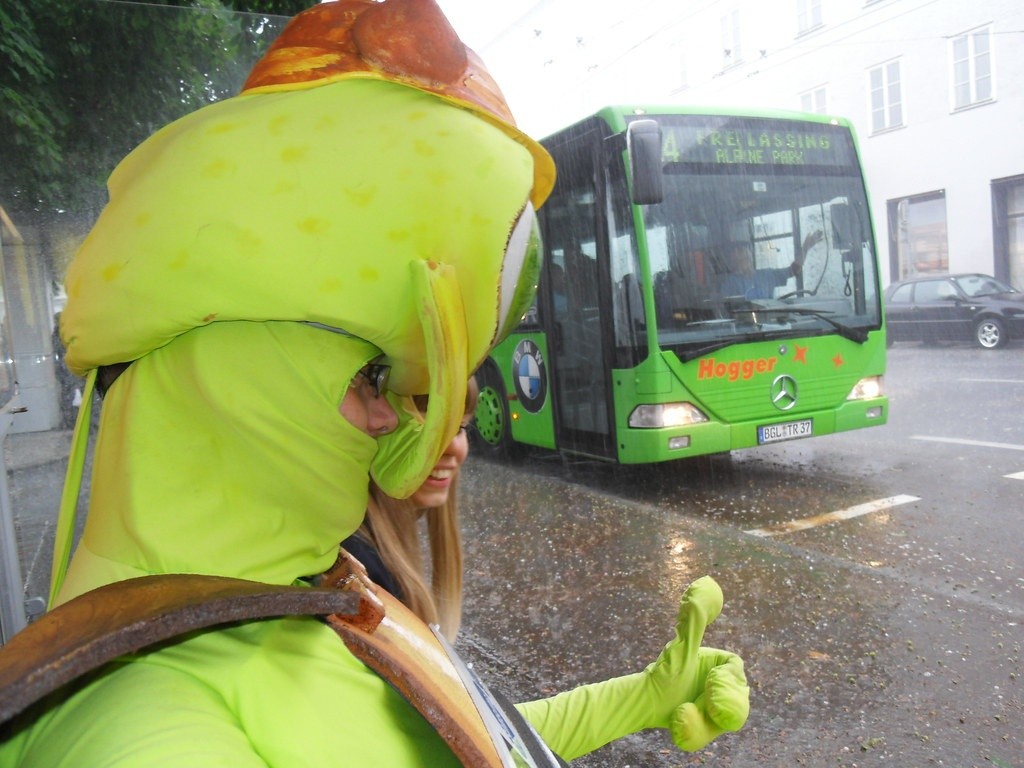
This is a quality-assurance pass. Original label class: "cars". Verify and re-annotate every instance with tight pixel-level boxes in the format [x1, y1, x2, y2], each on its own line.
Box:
[875, 273, 1023, 349]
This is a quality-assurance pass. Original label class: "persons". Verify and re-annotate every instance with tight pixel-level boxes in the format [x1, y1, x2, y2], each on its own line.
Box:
[1, 0, 750, 768]
[531, 263, 574, 312]
[49, 309, 80, 429]
[339, 371, 478, 645]
[718, 229, 823, 310]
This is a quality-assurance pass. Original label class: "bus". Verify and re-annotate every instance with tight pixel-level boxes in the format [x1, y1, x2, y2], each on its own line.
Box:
[464, 102, 893, 466]
